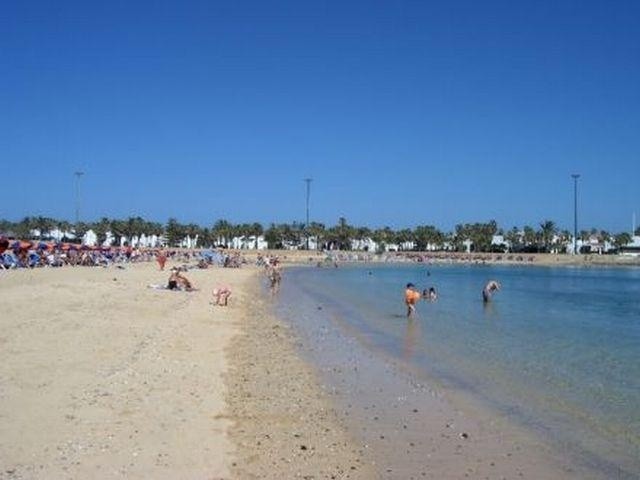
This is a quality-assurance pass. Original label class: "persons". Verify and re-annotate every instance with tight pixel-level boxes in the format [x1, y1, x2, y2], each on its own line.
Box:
[406, 283, 436, 317]
[0, 239, 287, 306]
[483, 281, 502, 303]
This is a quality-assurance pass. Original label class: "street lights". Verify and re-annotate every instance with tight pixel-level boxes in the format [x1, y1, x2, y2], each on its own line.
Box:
[303, 179, 313, 250]
[74, 171, 84, 226]
[571, 173, 580, 254]
